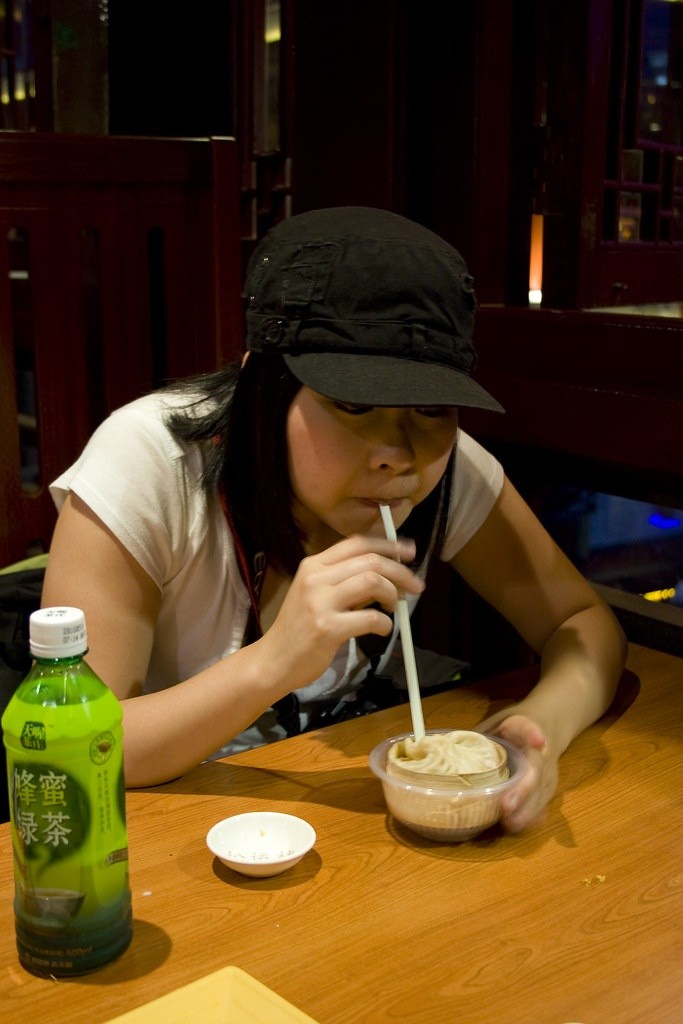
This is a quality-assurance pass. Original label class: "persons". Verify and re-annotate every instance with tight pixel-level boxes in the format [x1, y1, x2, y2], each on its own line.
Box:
[37, 200, 627, 831]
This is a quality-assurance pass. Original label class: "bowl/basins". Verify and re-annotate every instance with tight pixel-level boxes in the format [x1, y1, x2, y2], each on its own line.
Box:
[206, 812, 317, 878]
[369, 729, 528, 841]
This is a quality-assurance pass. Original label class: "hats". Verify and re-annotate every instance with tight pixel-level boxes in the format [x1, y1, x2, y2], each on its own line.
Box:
[244, 207, 506, 415]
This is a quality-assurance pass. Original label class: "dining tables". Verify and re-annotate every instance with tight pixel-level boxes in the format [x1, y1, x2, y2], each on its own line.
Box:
[1, 639, 683, 1023]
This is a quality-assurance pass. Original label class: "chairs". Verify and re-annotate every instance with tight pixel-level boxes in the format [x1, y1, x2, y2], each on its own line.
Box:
[1, 128, 240, 561]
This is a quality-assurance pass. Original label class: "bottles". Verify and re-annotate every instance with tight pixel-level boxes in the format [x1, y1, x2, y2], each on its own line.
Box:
[1, 605, 133, 977]
